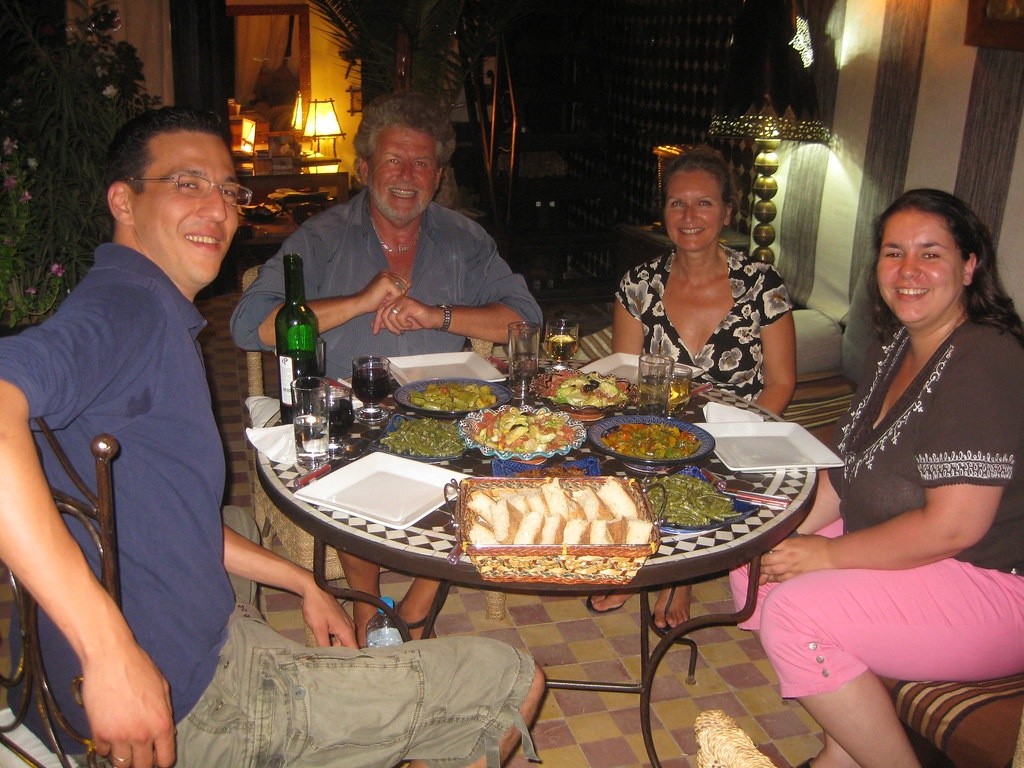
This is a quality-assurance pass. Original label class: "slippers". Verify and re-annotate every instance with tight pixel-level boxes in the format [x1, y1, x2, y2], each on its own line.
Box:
[585, 589, 627, 614]
[651, 586, 691, 634]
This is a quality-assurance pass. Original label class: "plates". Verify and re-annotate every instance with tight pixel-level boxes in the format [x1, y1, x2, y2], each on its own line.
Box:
[394, 377, 513, 417]
[293, 452, 475, 530]
[529, 367, 632, 421]
[369, 414, 470, 461]
[576, 352, 705, 386]
[691, 422, 845, 472]
[491, 457, 601, 477]
[459, 405, 587, 460]
[588, 415, 716, 473]
[380, 351, 506, 387]
[657, 467, 759, 535]
[236, 204, 278, 219]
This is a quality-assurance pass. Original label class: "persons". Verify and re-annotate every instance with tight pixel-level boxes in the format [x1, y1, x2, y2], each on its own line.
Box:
[230, 92, 546, 648]
[728, 189, 1024, 768]
[1, 105, 544, 768]
[585, 143, 796, 629]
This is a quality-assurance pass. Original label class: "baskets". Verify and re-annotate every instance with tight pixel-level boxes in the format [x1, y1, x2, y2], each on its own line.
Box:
[443, 478, 669, 586]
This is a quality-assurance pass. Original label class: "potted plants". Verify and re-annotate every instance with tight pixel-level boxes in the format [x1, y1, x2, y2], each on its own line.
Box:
[308, 0, 542, 237]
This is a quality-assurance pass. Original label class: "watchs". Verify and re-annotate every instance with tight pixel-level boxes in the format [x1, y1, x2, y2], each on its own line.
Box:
[434, 304, 455, 332]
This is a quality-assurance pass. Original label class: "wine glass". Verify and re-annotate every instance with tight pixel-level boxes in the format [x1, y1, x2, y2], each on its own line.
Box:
[351, 356, 390, 422]
[544, 318, 579, 374]
[329, 385, 354, 458]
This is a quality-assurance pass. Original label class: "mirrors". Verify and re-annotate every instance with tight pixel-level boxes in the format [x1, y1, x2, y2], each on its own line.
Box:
[229, 5, 312, 156]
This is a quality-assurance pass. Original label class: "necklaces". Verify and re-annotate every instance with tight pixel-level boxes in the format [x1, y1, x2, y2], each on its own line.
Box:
[366, 204, 421, 252]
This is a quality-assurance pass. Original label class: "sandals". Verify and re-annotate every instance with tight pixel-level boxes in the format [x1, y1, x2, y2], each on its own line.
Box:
[405, 614, 431, 629]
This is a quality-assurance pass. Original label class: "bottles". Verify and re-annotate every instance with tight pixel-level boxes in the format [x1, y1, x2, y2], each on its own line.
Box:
[276, 254, 318, 426]
[365, 597, 409, 648]
[228, 98, 242, 115]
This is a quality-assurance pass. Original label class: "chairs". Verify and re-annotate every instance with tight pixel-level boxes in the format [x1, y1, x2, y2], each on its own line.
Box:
[238, 268, 390, 618]
[0, 419, 122, 768]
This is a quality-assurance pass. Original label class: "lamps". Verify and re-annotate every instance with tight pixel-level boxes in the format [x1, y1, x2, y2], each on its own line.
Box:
[292, 91, 303, 146]
[707, 38, 833, 275]
[304, 99, 346, 157]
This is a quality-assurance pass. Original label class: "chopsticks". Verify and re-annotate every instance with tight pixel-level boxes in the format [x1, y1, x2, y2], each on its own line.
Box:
[337, 377, 352, 387]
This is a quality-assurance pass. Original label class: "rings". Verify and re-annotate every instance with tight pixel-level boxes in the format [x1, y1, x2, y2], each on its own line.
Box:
[391, 308, 399, 315]
[112, 754, 133, 761]
[395, 281, 400, 285]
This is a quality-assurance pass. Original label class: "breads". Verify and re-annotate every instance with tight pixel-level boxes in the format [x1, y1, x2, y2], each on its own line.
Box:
[467, 476, 654, 545]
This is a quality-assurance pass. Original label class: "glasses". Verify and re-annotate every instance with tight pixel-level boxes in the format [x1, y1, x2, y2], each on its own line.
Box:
[113, 174, 253, 207]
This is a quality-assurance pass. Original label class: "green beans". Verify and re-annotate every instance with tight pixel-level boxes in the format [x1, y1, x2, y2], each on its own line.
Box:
[380, 417, 468, 457]
[638, 472, 742, 526]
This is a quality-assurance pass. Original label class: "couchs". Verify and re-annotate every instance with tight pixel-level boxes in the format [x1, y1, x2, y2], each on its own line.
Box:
[576, 268, 1023, 768]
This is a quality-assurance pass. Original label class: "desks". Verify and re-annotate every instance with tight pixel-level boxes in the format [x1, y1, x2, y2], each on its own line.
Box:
[251, 360, 819, 768]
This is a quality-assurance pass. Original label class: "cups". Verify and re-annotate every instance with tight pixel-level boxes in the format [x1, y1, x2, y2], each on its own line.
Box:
[638, 354, 692, 416]
[291, 376, 330, 470]
[317, 337, 325, 376]
[508, 321, 541, 399]
